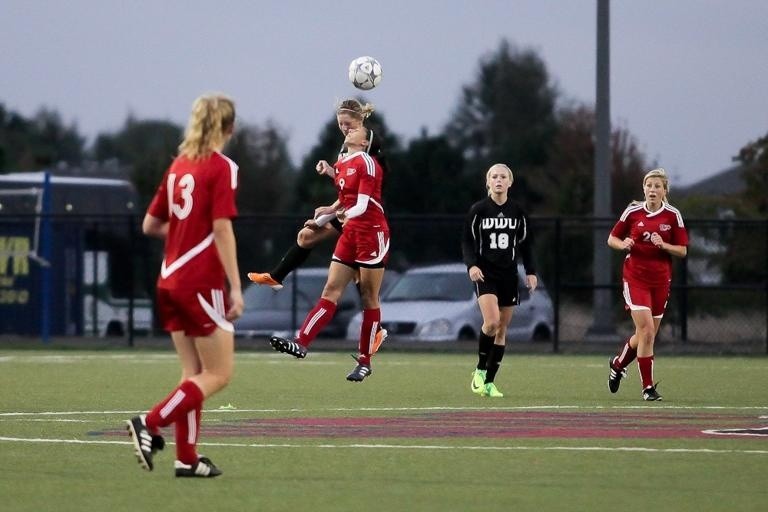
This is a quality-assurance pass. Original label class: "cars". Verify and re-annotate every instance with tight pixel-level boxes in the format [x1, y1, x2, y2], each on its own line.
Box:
[348, 260, 555, 347]
[231, 269, 400, 349]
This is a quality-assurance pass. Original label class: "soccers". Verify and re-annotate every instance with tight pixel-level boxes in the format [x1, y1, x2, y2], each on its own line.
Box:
[350, 57, 384, 89]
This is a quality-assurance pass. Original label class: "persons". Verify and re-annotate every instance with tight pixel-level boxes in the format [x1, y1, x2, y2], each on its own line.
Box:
[272, 126, 391, 382]
[607, 168, 688, 398]
[247, 99, 389, 358]
[455, 163, 537, 397]
[127, 96, 243, 477]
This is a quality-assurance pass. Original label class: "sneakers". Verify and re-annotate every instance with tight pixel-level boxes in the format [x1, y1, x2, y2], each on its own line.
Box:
[471, 367, 504, 398]
[347, 328, 388, 382]
[608, 356, 627, 393]
[174, 455, 221, 476]
[642, 381, 663, 401]
[247, 272, 284, 290]
[270, 337, 307, 358]
[126, 415, 164, 471]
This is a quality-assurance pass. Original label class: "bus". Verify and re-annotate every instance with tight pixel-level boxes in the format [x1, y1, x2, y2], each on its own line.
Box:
[0, 170, 159, 339]
